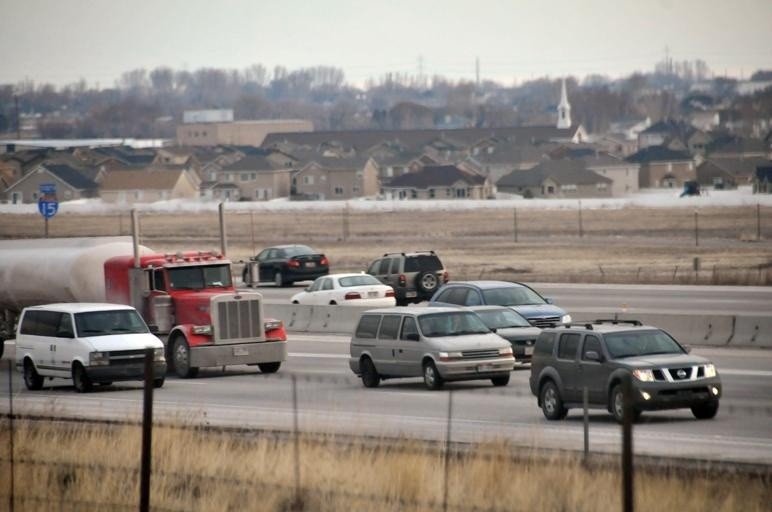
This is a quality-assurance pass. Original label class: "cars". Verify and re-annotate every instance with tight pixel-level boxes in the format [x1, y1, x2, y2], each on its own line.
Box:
[468, 305, 540, 364]
[242, 244, 329, 286]
[291, 273, 396, 308]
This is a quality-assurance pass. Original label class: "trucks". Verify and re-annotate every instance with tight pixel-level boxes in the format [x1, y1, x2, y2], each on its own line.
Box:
[0, 203, 289, 377]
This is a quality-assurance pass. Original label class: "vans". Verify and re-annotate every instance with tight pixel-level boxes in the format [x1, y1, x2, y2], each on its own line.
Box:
[14, 302, 167, 388]
[349, 305, 516, 389]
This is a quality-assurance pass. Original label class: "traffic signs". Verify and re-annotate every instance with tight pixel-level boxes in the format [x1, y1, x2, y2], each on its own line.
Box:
[39, 193, 58, 216]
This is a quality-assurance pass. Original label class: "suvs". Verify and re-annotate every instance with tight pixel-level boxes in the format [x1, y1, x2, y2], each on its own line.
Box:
[367, 251, 448, 305]
[431, 279, 571, 333]
[530, 319, 722, 425]
